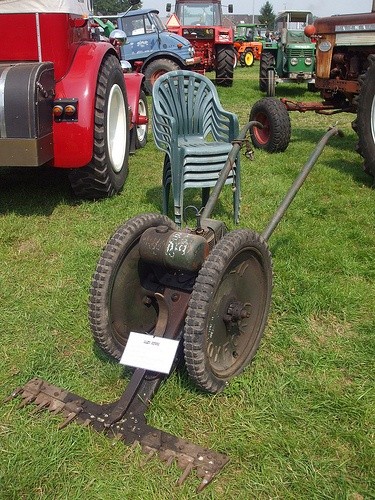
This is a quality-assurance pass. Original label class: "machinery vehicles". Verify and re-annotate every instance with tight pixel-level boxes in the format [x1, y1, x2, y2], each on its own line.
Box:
[0, 1, 375, 197]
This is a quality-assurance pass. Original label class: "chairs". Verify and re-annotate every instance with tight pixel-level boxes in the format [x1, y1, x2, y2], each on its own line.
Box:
[152, 70, 243, 223]
